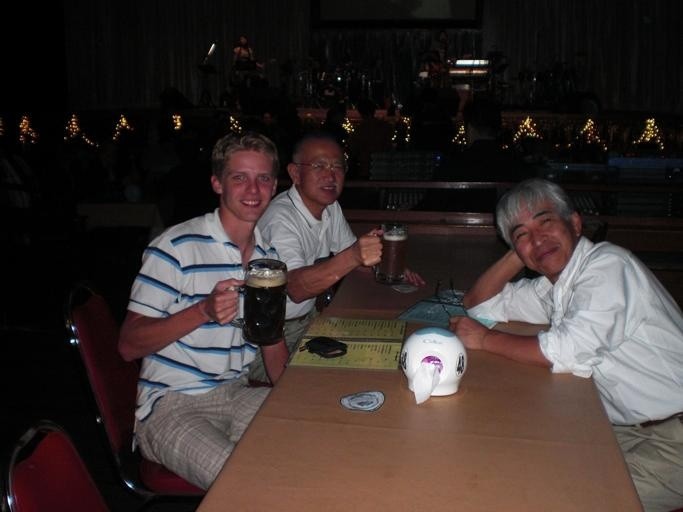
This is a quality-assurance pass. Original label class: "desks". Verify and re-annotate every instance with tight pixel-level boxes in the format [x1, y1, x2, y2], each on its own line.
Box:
[197, 234, 644, 511]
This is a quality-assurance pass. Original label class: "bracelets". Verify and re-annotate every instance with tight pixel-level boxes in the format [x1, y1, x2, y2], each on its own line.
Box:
[199, 302, 211, 320]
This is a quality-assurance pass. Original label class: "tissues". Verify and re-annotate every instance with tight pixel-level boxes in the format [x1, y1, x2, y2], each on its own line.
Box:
[398, 325, 468, 405]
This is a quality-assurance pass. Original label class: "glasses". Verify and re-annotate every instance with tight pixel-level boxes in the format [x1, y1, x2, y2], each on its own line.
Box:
[293, 159, 349, 174]
[434, 277, 470, 318]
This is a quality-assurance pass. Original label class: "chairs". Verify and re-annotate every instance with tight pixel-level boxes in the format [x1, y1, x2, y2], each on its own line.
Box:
[6, 420, 108, 511]
[66, 283, 203, 510]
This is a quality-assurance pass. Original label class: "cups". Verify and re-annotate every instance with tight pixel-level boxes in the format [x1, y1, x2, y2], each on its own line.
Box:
[225, 258, 288, 346]
[370, 221, 410, 286]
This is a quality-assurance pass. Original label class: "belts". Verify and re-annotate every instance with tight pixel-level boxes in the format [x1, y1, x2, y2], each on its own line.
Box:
[612, 411, 683, 428]
[285, 311, 311, 322]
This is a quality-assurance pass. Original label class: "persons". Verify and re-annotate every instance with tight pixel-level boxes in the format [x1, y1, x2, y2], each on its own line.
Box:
[445, 176, 679, 512]
[117, 131, 288, 491]
[247, 129, 427, 384]
[155, 49, 618, 212]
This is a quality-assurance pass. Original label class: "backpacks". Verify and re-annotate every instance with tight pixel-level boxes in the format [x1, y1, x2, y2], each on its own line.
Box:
[314, 252, 342, 311]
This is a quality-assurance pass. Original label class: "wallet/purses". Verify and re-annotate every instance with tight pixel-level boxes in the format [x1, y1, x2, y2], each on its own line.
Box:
[298, 336, 348, 358]
